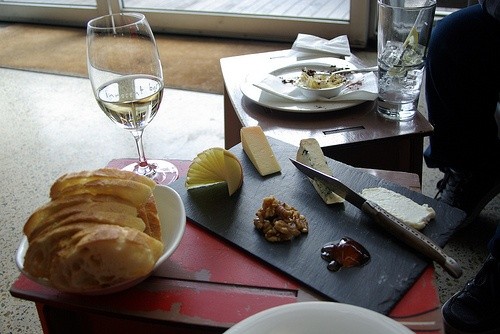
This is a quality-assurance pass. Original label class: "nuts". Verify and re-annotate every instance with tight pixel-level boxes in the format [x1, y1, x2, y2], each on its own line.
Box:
[254, 197, 309, 243]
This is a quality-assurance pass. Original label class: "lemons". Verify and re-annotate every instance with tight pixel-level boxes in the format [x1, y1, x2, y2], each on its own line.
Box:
[389, 27, 418, 75]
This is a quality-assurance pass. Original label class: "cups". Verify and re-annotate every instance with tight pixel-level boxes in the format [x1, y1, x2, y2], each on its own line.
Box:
[374, 0, 438, 122]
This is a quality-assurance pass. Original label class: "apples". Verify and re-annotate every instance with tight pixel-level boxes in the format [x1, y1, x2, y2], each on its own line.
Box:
[185, 147, 243, 198]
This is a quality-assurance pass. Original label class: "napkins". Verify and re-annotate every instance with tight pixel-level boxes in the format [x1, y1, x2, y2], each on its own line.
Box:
[292, 34, 351, 60]
[252, 72, 378, 100]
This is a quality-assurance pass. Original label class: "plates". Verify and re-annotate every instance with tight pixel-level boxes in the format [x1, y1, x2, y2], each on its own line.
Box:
[221, 301, 418, 334]
[239, 57, 371, 112]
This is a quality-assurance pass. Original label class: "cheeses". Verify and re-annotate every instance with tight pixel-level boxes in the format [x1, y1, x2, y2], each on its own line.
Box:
[240, 125, 282, 176]
[296, 138, 345, 205]
[360, 187, 436, 231]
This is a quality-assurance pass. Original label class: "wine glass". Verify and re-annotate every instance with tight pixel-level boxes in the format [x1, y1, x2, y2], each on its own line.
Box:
[85, 13, 179, 186]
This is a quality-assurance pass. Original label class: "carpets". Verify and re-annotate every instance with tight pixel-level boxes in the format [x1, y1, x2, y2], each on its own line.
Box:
[0, 22, 430, 122]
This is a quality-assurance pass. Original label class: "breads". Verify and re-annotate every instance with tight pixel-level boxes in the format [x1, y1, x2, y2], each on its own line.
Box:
[23, 168, 164, 290]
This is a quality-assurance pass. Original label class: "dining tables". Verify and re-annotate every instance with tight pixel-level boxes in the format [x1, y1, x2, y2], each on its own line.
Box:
[9, 156, 444, 334]
[219, 53, 435, 190]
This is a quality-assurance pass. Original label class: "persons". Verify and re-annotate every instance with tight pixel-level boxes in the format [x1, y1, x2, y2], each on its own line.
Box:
[425, 0, 500, 334]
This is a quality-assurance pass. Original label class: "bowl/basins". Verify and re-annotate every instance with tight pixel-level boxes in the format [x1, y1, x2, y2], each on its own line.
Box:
[297, 71, 347, 96]
[15, 183, 187, 294]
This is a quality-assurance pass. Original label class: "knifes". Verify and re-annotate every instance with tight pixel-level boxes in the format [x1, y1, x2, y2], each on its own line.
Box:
[288, 157, 463, 279]
